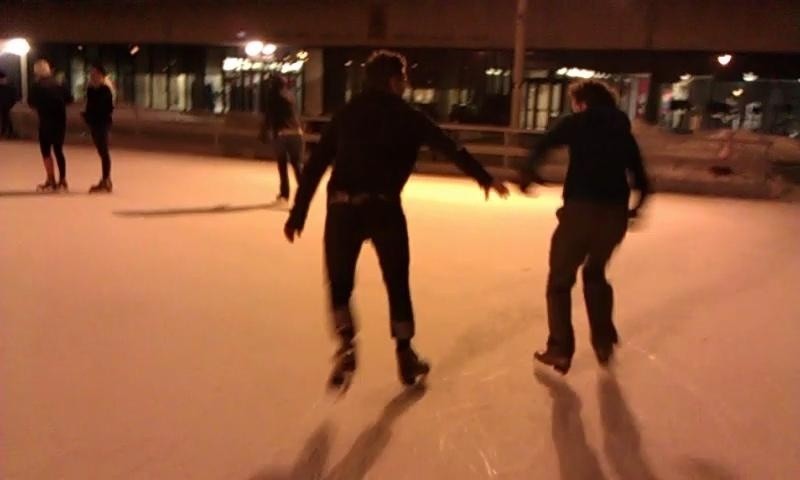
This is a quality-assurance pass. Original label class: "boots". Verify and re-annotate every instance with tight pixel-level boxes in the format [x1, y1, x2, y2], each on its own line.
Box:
[534, 344, 574, 374]
[396, 349, 431, 384]
[324, 343, 358, 391]
[594, 347, 613, 366]
[89, 180, 113, 192]
[38, 178, 69, 190]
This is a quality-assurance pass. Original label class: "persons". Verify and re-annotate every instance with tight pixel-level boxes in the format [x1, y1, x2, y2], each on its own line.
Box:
[0, 70, 18, 136]
[518, 80, 652, 375]
[260, 74, 303, 202]
[28, 58, 68, 193]
[284, 50, 510, 387]
[86, 63, 112, 193]
[191, 70, 217, 110]
[669, 71, 741, 134]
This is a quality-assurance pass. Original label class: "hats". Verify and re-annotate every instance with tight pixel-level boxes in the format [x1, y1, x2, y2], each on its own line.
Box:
[92, 63, 109, 76]
[33, 59, 53, 77]
